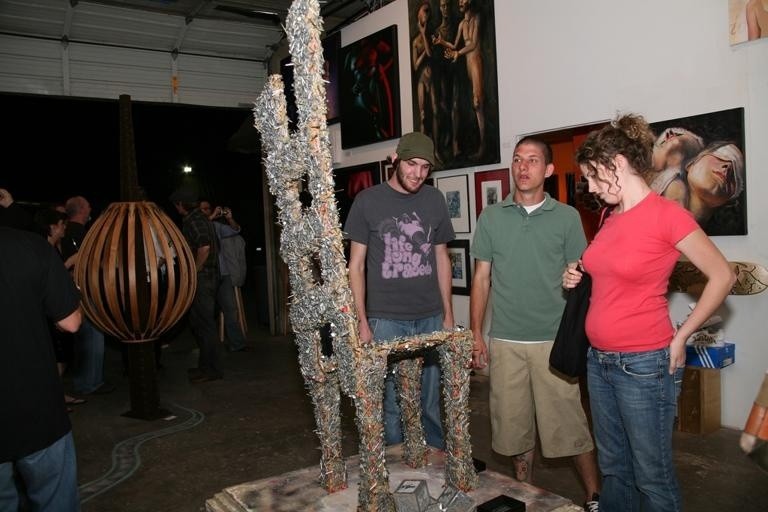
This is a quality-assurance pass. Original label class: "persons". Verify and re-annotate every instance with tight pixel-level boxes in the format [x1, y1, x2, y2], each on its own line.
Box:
[746, 0, 768, 41]
[342, 133, 456, 452]
[470, 135, 601, 511]
[643, 126, 703, 212]
[344, 37, 395, 139]
[447, 192, 459, 218]
[3, 189, 251, 412]
[1, 224, 85, 512]
[686, 140, 746, 224]
[413, 0, 486, 171]
[566, 113, 738, 512]
[391, 211, 429, 245]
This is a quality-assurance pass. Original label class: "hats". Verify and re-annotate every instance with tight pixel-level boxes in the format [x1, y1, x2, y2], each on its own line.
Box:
[396, 132, 436, 167]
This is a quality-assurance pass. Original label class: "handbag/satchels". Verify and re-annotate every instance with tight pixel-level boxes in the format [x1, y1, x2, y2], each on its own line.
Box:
[548, 267, 590, 378]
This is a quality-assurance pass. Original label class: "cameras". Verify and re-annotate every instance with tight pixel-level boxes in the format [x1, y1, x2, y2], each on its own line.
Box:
[217, 208, 228, 219]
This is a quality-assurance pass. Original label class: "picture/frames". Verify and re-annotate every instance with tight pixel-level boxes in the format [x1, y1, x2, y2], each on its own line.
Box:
[474, 168, 510, 222]
[381, 160, 392, 183]
[436, 174, 470, 233]
[447, 240, 471, 295]
